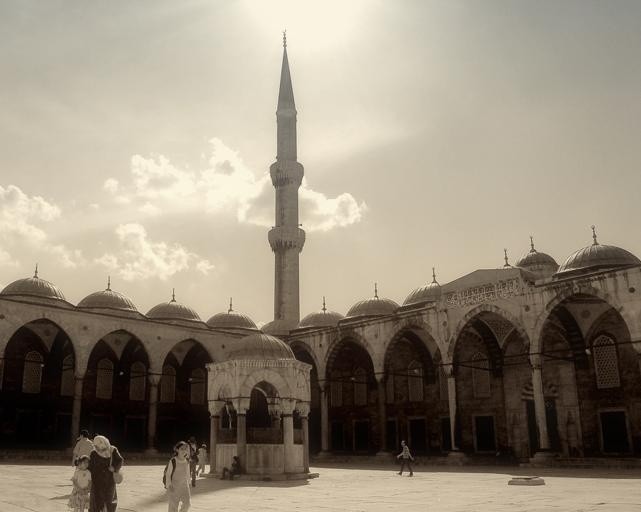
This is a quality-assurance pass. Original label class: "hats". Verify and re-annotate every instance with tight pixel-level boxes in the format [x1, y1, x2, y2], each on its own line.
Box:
[95, 436, 110, 449]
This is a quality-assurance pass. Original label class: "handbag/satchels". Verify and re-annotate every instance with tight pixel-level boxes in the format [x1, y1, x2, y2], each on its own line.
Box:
[112, 471, 122, 484]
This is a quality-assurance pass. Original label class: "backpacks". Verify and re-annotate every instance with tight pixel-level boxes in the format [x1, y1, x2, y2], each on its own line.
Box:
[163, 457, 176, 485]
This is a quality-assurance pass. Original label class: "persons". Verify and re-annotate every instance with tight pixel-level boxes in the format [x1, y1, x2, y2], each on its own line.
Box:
[195, 443, 207, 476]
[187, 437, 200, 487]
[67, 455, 92, 512]
[220, 456, 242, 480]
[395, 440, 415, 476]
[71, 429, 95, 470]
[87, 434, 124, 512]
[165, 441, 195, 512]
